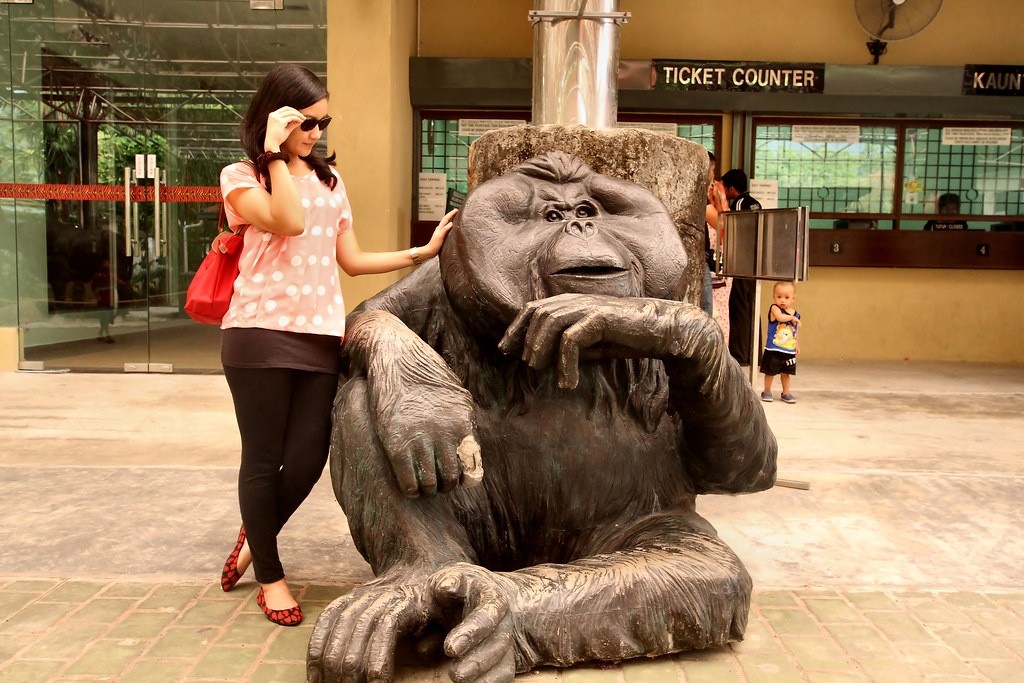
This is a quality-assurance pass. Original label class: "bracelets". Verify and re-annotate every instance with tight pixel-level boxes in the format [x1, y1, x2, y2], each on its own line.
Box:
[217, 60, 459, 630]
[410, 246, 423, 267]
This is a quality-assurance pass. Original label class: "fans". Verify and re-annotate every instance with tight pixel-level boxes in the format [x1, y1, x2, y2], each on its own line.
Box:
[853, 0, 943, 65]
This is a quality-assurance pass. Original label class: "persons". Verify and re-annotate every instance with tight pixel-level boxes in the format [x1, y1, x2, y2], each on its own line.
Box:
[923, 191, 968, 231]
[759, 279, 802, 403]
[703, 149, 763, 369]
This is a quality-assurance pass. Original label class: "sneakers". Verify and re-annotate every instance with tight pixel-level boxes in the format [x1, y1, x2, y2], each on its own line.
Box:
[760, 392, 773, 402]
[780, 392, 797, 404]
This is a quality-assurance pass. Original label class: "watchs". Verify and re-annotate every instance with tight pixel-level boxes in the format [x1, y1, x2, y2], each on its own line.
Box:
[256, 150, 290, 168]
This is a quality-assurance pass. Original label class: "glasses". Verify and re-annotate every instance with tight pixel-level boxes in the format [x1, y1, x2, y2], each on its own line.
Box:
[300, 117, 332, 131]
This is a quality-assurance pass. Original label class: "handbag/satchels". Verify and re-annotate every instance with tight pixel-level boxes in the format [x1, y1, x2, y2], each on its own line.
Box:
[183, 231, 245, 326]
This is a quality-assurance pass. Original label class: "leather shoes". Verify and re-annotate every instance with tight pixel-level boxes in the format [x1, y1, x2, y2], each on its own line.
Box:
[221, 522, 247, 592]
[255, 587, 304, 626]
